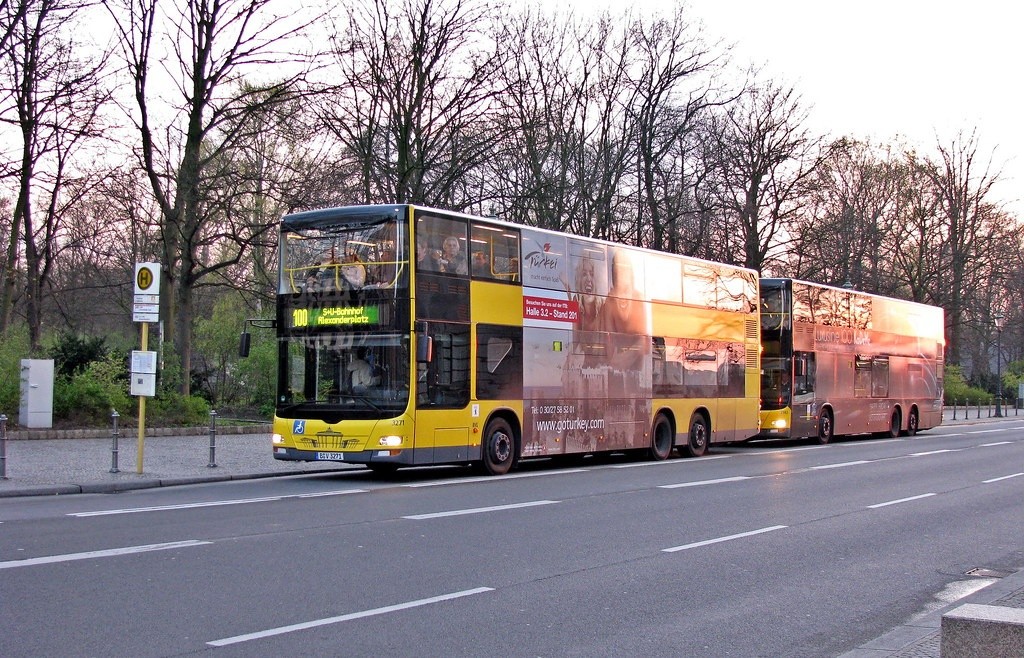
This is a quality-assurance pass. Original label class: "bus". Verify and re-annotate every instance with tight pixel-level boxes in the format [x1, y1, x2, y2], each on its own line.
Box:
[238, 204, 761, 476]
[757, 277, 945, 446]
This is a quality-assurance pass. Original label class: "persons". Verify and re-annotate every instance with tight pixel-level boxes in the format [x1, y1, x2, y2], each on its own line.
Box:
[419, 362, 438, 402]
[471, 249, 494, 277]
[417, 240, 439, 271]
[305, 241, 396, 291]
[441, 236, 467, 274]
[347, 347, 380, 395]
[777, 373, 791, 391]
[508, 258, 518, 281]
[573, 253, 644, 370]
